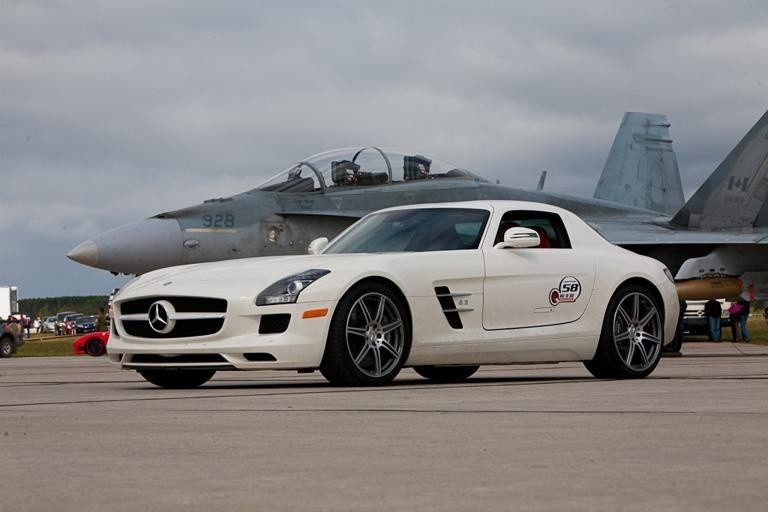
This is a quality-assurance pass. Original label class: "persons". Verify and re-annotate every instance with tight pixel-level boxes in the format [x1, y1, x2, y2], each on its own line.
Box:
[704, 283, 768, 342]
[0, 307, 107, 332]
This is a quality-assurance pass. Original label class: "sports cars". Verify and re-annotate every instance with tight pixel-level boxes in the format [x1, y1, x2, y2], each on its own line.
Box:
[98, 193, 686, 396]
[68, 330, 111, 358]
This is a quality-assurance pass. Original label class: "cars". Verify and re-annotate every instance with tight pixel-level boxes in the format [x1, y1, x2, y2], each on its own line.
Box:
[36, 310, 98, 336]
[0, 315, 25, 358]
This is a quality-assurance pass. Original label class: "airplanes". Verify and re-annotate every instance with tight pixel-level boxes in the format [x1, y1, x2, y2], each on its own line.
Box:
[61, 107, 767, 359]
